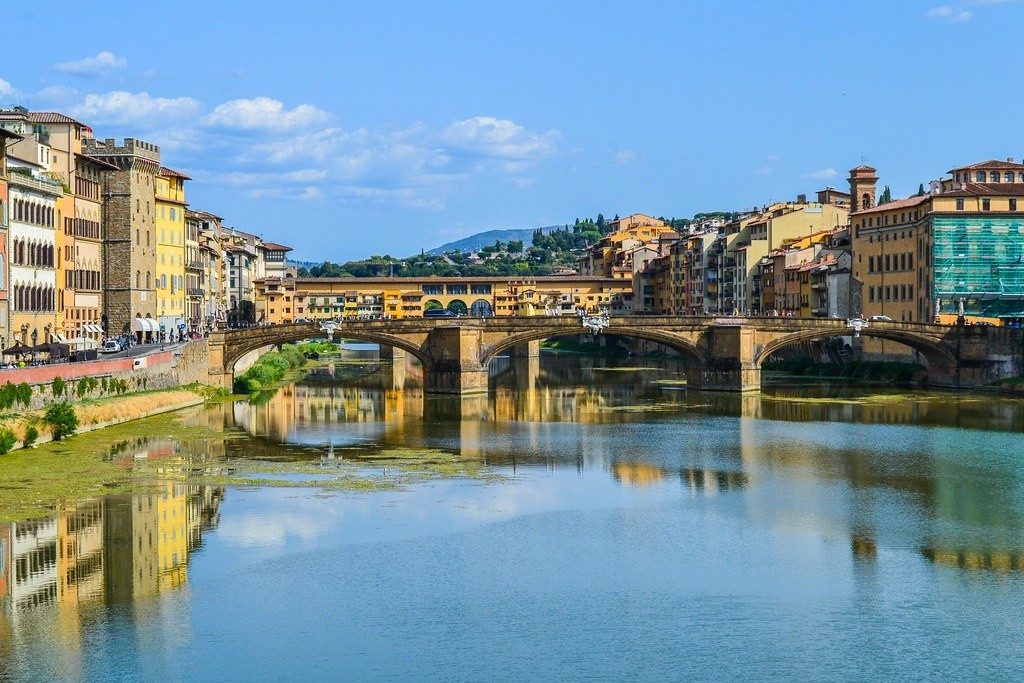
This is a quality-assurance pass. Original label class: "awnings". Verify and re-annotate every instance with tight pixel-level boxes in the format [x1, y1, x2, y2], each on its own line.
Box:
[136, 318, 161, 331]
[82, 325, 105, 333]
[51, 333, 66, 343]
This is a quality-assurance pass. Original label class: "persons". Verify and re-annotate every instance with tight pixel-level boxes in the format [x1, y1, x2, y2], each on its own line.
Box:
[704, 308, 797, 316]
[259, 313, 392, 328]
[101, 333, 138, 352]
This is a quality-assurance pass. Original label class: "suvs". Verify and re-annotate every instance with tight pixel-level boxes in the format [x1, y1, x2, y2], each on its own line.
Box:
[424, 310, 457, 317]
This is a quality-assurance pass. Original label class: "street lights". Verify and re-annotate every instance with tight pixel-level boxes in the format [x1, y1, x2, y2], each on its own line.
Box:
[83, 331, 87, 361]
[30, 333, 36, 347]
[126, 329, 129, 357]
[330, 303, 333, 321]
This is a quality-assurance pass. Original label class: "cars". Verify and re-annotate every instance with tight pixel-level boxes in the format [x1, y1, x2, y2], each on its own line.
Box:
[103, 341, 120, 352]
[871, 315, 893, 320]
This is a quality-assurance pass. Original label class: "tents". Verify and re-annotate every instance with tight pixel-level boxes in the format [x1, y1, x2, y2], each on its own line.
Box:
[2, 340, 71, 364]
